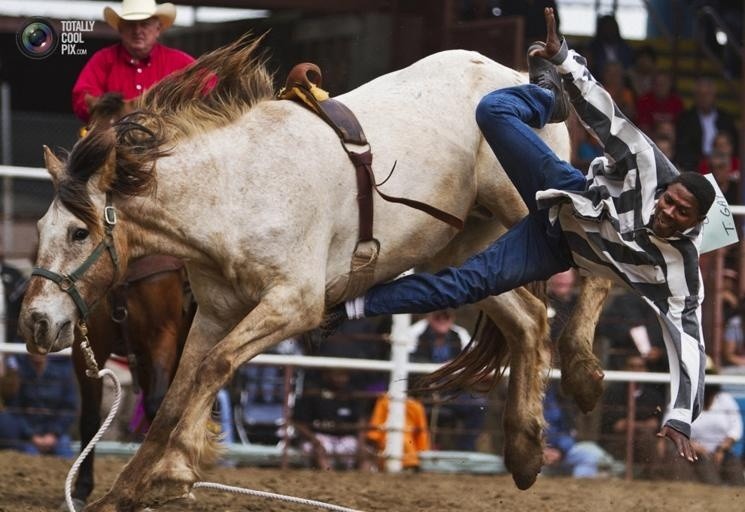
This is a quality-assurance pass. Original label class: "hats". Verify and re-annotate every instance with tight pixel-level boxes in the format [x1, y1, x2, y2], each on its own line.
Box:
[104, 0, 176, 32]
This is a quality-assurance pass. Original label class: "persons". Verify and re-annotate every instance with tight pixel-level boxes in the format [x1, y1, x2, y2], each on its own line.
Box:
[0, 7, 745, 484]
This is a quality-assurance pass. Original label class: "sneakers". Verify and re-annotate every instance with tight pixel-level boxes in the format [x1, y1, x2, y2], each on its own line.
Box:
[304, 301, 349, 354]
[527, 41, 569, 123]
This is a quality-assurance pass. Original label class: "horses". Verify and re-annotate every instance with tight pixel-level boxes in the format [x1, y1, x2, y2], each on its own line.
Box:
[14, 28, 612, 511]
[57, 92, 197, 511]
